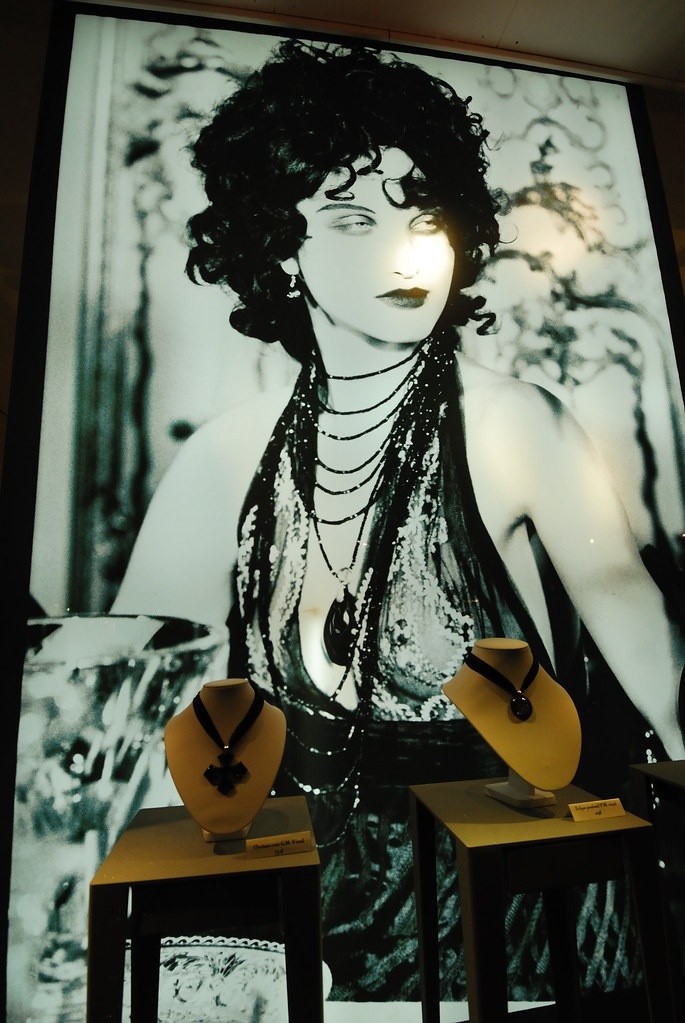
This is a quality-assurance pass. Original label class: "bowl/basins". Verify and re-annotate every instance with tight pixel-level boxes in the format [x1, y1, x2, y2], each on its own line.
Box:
[76, 936, 334, 1023]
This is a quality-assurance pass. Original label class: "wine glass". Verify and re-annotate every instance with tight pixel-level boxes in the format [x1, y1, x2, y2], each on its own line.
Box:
[6, 610, 230, 1023]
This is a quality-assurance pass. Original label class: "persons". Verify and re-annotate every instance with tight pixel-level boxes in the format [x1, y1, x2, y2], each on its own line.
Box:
[110, 45, 685, 1002]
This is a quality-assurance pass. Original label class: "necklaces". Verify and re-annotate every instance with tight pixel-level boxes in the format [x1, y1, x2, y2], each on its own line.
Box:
[236, 334, 455, 848]
[465, 649, 542, 719]
[193, 690, 265, 795]
